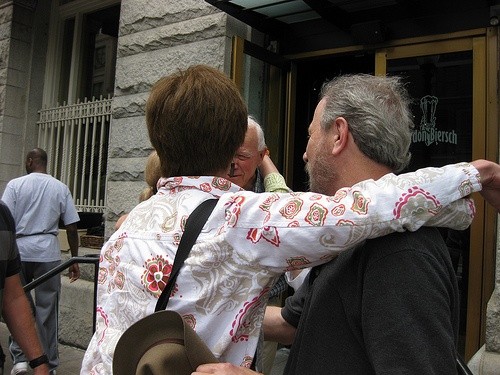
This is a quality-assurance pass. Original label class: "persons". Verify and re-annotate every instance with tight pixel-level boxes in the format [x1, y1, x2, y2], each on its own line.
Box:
[80, 65, 500, 375]
[188, 73, 456, 375]
[113, 115, 313, 375]
[0, 199, 51, 375]
[0, 147, 80, 375]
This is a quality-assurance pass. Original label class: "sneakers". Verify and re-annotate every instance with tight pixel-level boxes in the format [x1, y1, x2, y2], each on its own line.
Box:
[10, 362, 28, 375]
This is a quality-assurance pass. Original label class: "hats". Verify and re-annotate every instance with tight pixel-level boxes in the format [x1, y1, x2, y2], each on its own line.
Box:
[112, 310, 218, 375]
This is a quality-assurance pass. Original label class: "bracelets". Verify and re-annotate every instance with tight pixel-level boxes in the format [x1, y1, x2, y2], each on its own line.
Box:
[30, 355, 49, 369]
